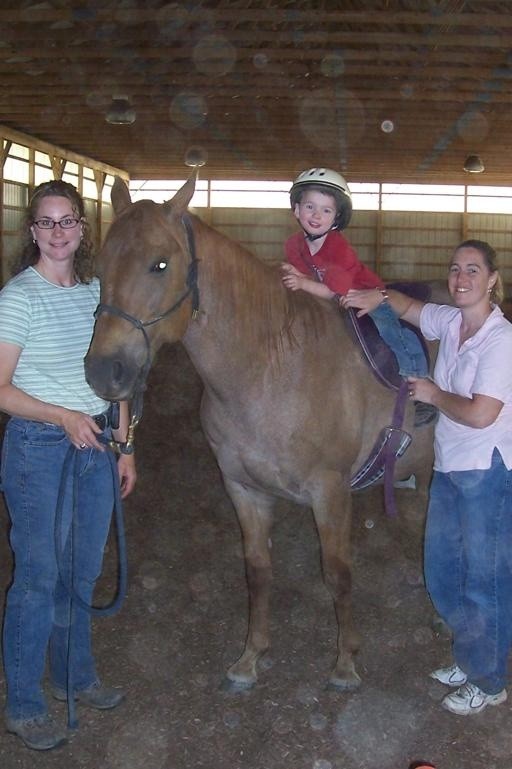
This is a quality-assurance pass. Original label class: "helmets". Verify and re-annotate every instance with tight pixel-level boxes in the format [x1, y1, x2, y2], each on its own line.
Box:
[290, 167, 351, 229]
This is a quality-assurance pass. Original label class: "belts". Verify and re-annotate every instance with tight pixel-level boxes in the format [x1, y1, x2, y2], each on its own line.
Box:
[91, 413, 111, 431]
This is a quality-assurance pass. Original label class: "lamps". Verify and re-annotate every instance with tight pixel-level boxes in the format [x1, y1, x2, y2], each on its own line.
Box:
[103, 90, 139, 128]
[462, 152, 485, 175]
[183, 139, 210, 171]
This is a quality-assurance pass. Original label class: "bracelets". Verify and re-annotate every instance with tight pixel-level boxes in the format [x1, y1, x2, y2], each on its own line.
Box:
[375, 285, 390, 305]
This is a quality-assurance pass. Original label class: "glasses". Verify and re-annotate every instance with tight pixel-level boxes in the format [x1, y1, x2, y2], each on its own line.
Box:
[35, 219, 80, 229]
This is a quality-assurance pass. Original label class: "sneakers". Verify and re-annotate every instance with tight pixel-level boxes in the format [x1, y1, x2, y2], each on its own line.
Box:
[413, 400, 438, 429]
[441, 681, 508, 717]
[52, 677, 124, 710]
[428, 664, 468, 687]
[4, 709, 68, 751]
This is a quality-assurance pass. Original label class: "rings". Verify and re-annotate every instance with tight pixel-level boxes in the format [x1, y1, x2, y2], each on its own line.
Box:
[409, 389, 414, 396]
[80, 443, 86, 449]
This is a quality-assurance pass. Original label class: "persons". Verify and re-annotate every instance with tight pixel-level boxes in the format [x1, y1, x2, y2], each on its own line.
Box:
[339, 238, 511, 719]
[279, 166, 438, 427]
[2, 178, 140, 752]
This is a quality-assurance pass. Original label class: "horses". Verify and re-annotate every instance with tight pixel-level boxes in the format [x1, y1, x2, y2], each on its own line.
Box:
[81, 172, 512, 694]
